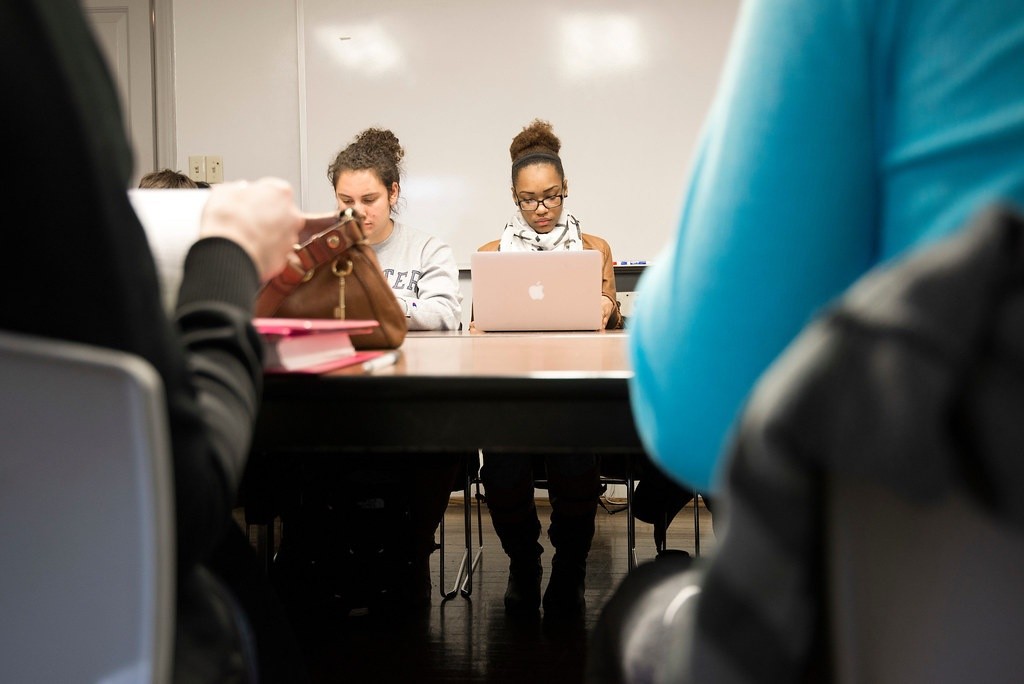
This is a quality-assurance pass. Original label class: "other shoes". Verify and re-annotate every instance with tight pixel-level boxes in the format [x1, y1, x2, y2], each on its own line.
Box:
[541, 554, 591, 623]
[503, 548, 543, 617]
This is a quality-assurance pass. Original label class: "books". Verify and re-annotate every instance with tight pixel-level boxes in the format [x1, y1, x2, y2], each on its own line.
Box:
[250, 319, 385, 374]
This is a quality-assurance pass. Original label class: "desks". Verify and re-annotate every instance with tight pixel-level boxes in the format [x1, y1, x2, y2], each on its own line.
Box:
[248, 331, 652, 455]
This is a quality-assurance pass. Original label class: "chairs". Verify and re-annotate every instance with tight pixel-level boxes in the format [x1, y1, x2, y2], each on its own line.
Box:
[678, 204, 1024, 684]
[0, 339, 171, 684]
[461, 299, 637, 599]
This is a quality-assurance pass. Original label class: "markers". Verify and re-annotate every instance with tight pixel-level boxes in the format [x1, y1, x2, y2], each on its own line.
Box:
[612, 261, 652, 265]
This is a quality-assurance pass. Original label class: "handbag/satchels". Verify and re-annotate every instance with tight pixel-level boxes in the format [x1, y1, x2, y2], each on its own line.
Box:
[252, 212, 407, 351]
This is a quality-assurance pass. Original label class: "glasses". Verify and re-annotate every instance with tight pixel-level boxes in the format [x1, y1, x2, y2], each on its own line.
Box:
[514, 180, 565, 211]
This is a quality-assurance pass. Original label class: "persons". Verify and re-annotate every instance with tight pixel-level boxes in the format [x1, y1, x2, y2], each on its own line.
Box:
[139, 169, 211, 189]
[597, 0, 1024, 681]
[0, 0, 306, 683]
[468, 121, 624, 622]
[326, 130, 464, 593]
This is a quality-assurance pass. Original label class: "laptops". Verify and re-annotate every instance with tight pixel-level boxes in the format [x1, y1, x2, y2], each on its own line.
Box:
[471, 249, 602, 330]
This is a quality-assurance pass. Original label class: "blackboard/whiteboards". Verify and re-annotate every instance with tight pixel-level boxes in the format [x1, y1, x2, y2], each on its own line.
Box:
[298, 1, 747, 275]
